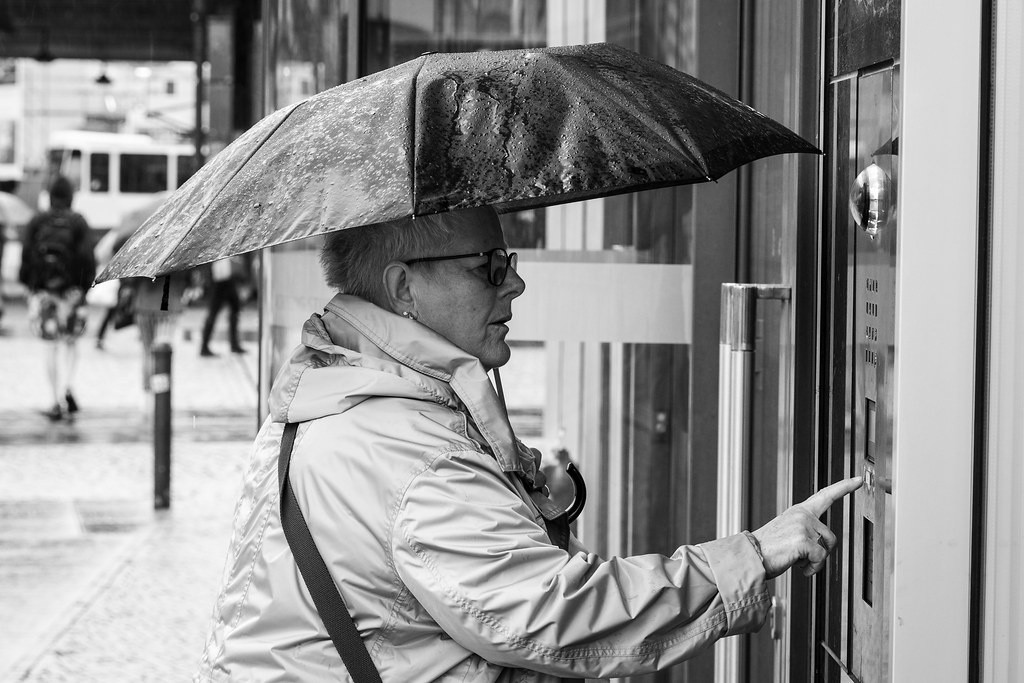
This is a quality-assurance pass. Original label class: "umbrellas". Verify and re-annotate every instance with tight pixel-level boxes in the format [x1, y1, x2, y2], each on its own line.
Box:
[91, 44, 828, 532]
[0, 191, 36, 226]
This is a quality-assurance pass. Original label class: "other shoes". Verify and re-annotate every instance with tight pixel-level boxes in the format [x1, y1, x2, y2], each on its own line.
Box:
[200, 348, 212, 355]
[65, 393, 76, 411]
[51, 405, 61, 416]
[232, 346, 245, 352]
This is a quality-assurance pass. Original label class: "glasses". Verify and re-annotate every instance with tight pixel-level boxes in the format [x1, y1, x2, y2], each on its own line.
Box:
[404, 247, 518, 286]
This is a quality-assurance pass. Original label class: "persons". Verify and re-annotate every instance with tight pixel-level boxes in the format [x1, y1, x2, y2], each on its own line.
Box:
[0, 174, 258, 422]
[192, 205, 863, 683]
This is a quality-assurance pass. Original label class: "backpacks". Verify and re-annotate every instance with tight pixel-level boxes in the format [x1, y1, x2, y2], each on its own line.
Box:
[34, 212, 83, 291]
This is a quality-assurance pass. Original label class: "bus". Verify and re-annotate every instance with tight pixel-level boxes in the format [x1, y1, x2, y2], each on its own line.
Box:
[36, 131, 210, 236]
